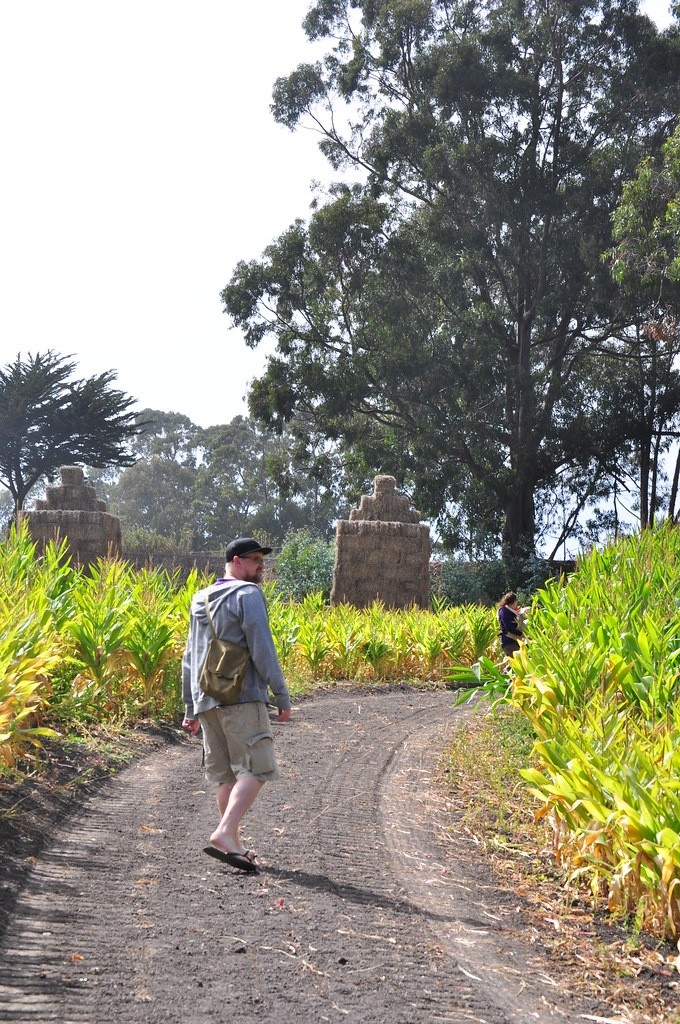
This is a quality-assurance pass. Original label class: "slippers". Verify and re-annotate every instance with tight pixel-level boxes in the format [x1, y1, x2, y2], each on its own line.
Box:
[203, 847, 260, 871]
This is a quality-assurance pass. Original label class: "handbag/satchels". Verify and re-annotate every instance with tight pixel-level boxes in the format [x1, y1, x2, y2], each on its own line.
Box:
[199, 637, 269, 705]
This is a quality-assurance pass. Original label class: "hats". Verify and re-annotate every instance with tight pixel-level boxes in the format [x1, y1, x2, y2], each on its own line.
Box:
[226, 538, 272, 561]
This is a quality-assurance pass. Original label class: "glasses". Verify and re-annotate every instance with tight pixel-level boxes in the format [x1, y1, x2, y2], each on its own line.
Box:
[239, 555, 264, 563]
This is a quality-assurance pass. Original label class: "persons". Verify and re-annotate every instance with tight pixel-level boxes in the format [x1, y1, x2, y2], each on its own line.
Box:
[179, 538, 291, 873]
[498, 592, 522, 663]
